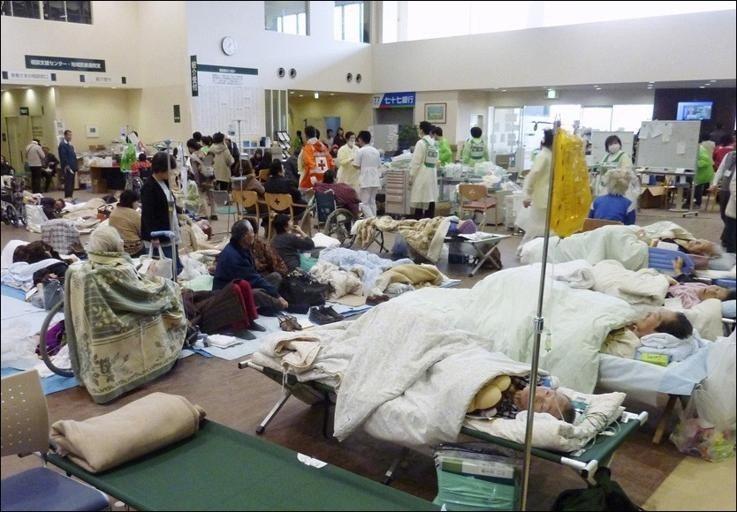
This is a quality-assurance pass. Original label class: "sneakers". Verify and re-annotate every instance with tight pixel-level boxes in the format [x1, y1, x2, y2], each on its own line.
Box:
[319, 305, 343, 321]
[308, 307, 333, 324]
[364, 294, 390, 307]
[279, 314, 303, 332]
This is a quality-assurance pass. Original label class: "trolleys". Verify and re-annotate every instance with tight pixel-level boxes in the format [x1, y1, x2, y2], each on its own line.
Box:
[383, 167, 412, 219]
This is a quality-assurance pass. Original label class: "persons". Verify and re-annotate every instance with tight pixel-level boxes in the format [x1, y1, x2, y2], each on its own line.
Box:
[407, 121, 489, 219]
[363, 231, 736, 424]
[693, 128, 737, 251]
[523, 128, 556, 227]
[1, 127, 381, 339]
[591, 135, 638, 227]
[394, 216, 476, 236]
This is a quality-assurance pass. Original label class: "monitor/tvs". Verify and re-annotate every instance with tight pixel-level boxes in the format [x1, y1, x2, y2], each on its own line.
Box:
[675, 100, 715, 121]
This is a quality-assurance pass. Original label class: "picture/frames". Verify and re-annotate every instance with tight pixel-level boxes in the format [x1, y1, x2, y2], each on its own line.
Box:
[423, 102, 447, 125]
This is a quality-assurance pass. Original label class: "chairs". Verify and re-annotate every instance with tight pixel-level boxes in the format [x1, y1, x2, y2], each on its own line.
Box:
[263, 191, 313, 242]
[663, 174, 678, 206]
[207, 187, 239, 236]
[458, 182, 499, 231]
[705, 186, 723, 214]
[581, 218, 624, 231]
[2, 369, 111, 512]
[230, 190, 280, 242]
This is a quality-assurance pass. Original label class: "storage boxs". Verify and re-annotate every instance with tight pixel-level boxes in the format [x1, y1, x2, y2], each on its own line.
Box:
[91, 178, 108, 193]
[639, 184, 667, 209]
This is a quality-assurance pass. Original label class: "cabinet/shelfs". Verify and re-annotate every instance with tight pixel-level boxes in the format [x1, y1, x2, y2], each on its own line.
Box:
[275, 130, 292, 168]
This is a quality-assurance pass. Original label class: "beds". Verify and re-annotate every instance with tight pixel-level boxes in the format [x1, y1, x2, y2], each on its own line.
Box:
[493, 298, 716, 444]
[18, 421, 453, 512]
[639, 294, 737, 337]
[239, 320, 650, 489]
[365, 220, 511, 277]
[517, 241, 736, 293]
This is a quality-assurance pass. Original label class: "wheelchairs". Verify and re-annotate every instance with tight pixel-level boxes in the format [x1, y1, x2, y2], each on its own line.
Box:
[309, 182, 384, 254]
[0, 175, 26, 227]
[24, 260, 213, 383]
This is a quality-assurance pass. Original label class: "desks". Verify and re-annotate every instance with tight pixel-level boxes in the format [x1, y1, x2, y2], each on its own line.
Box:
[436, 172, 513, 203]
[88, 165, 136, 191]
